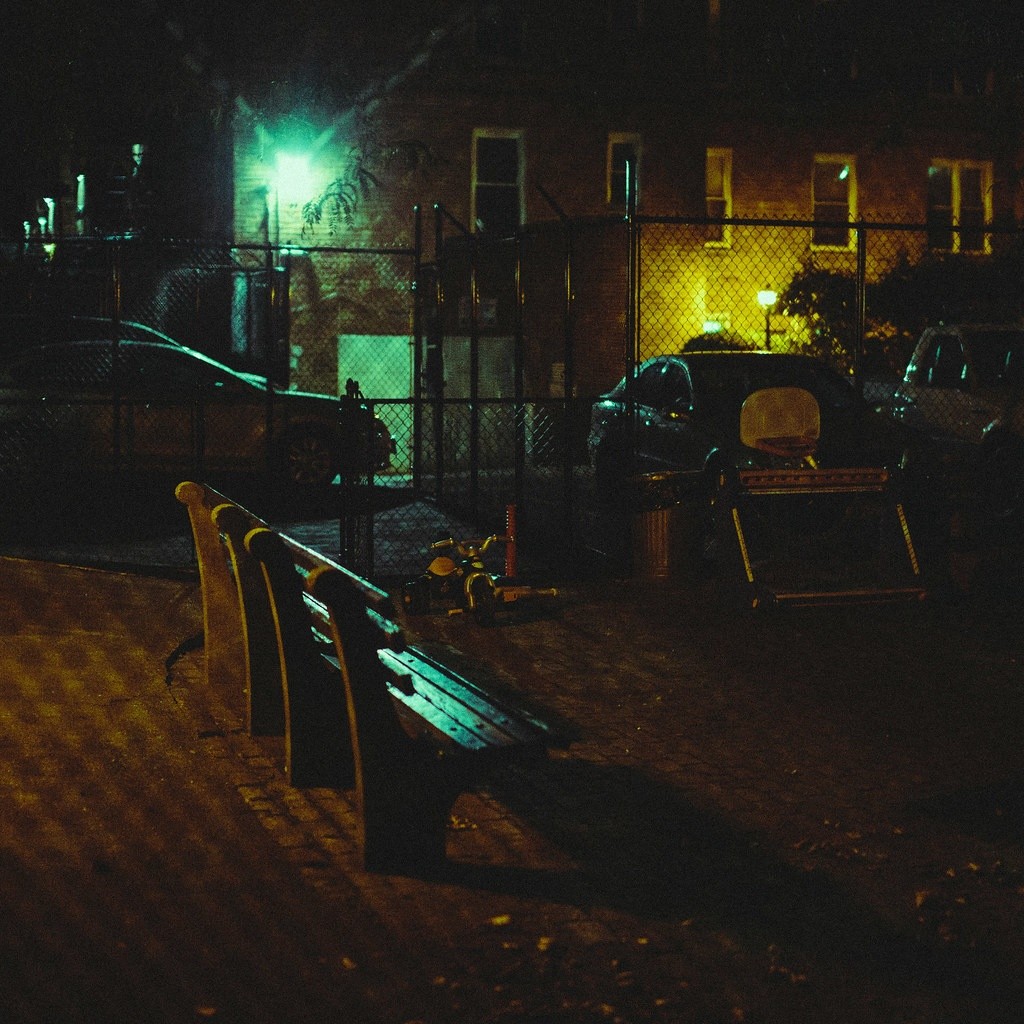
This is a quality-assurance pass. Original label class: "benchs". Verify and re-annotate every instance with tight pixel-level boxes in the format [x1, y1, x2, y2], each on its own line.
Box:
[175, 481, 573, 873]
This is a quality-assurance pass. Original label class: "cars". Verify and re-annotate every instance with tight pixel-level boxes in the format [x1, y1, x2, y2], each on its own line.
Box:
[0, 338, 396, 498]
[891, 324, 1024, 445]
[0, 312, 268, 391]
[586, 349, 945, 548]
